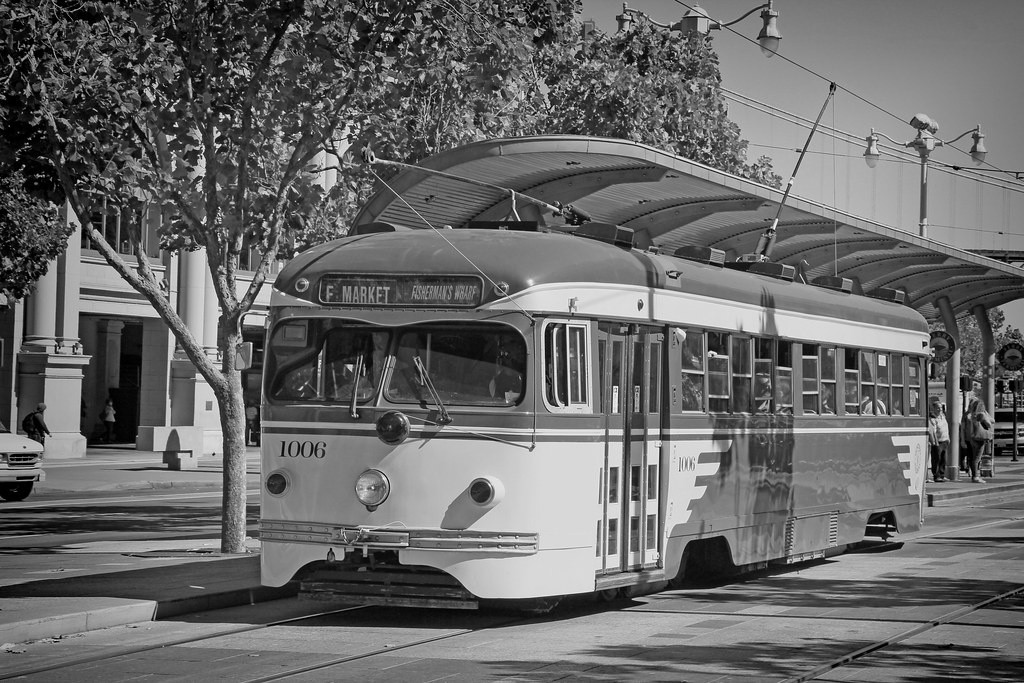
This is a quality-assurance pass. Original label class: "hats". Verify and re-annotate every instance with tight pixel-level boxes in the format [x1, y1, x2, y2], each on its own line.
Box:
[36, 403, 47, 412]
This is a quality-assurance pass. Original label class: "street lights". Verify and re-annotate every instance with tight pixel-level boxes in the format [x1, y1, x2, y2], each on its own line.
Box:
[862, 115, 988, 238]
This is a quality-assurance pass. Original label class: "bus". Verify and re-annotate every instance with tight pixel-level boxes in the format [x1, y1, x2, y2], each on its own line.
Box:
[229, 125, 929, 615]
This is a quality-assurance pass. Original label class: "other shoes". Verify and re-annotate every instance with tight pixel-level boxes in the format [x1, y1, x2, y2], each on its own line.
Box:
[942, 476, 950, 482]
[934, 477, 944, 482]
[972, 477, 985, 483]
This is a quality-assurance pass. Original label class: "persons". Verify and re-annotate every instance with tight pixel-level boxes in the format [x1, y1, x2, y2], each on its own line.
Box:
[246, 399, 259, 447]
[28, 402, 52, 447]
[103, 399, 116, 445]
[681, 347, 921, 415]
[960, 398, 995, 484]
[928, 401, 951, 483]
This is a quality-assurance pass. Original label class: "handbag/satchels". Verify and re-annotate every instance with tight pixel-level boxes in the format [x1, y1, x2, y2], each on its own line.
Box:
[973, 421, 994, 440]
[98, 411, 107, 421]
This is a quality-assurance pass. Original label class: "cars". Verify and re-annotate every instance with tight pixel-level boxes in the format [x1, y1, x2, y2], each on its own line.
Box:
[0, 422, 45, 502]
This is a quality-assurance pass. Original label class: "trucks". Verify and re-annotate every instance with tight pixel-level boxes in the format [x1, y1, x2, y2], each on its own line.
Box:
[929, 381, 981, 415]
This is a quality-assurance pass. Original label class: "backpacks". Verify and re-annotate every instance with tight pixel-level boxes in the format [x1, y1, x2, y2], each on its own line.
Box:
[22, 411, 41, 435]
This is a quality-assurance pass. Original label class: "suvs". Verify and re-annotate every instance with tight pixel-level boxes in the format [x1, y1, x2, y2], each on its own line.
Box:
[993, 407, 1024, 457]
